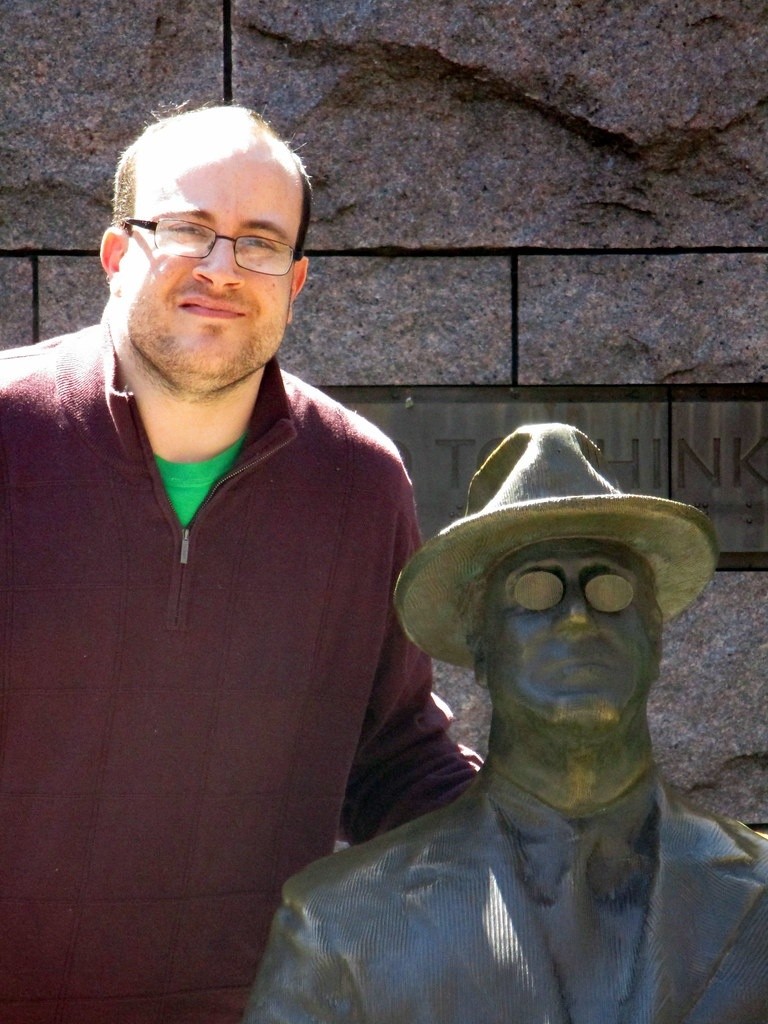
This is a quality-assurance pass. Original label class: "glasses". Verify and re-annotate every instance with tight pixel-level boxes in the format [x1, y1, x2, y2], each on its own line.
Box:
[117, 217, 298, 277]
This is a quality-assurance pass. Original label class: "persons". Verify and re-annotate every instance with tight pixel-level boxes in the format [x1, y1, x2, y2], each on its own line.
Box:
[244, 420, 768, 1024]
[1, 105, 486, 1024]
